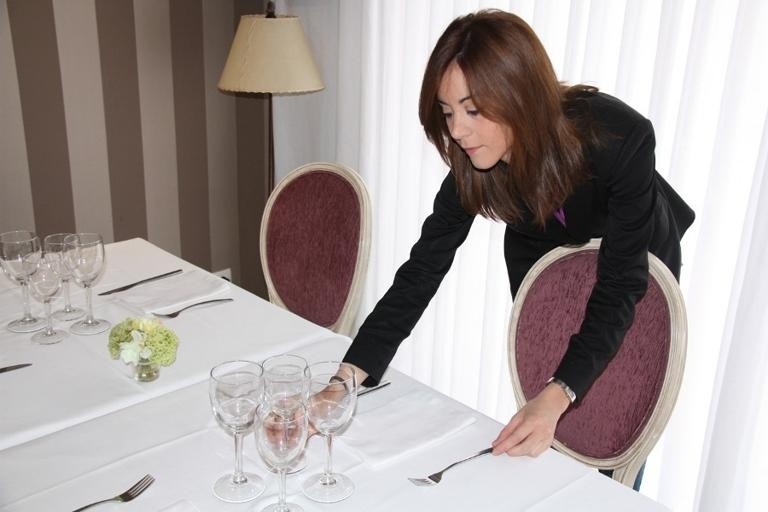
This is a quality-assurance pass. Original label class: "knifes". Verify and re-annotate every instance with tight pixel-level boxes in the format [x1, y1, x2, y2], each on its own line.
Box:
[0, 363, 32, 373]
[98, 269, 184, 297]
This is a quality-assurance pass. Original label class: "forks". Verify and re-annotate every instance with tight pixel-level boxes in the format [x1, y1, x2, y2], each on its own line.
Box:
[69, 473, 156, 511]
[407, 448, 493, 489]
[152, 298, 234, 321]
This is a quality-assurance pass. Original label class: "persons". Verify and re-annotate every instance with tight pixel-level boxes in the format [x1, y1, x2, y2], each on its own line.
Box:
[263, 6, 697, 494]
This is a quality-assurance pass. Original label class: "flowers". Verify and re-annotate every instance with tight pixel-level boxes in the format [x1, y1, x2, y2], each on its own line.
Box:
[106, 314, 179, 377]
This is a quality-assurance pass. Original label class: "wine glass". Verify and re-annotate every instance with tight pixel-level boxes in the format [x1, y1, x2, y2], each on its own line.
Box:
[0, 231, 112, 345]
[208, 354, 358, 512]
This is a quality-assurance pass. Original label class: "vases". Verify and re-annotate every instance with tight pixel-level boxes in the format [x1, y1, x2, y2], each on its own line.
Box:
[135, 356, 158, 381]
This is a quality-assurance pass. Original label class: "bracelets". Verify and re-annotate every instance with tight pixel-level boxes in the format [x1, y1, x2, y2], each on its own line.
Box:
[329, 376, 352, 391]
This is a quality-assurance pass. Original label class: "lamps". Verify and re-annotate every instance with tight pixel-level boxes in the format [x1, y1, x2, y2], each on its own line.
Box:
[218, 3, 326, 198]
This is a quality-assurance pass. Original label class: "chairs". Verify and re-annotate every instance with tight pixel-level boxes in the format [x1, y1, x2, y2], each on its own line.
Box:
[507, 244, 691, 493]
[257, 161, 372, 343]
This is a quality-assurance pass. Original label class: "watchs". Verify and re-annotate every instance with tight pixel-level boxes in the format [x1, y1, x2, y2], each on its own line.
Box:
[544, 374, 577, 405]
